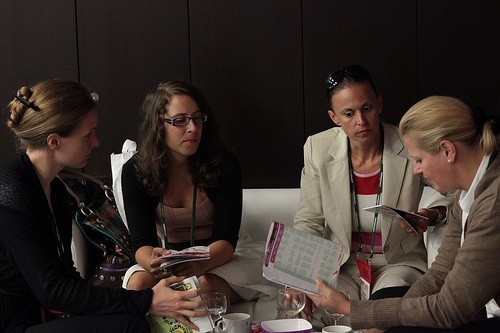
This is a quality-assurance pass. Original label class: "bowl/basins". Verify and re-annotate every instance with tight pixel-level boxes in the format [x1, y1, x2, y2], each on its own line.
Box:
[260, 318, 312, 333]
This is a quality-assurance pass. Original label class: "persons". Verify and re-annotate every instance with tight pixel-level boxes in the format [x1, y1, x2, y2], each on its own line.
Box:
[275, 64, 461, 332]
[306, 96, 500, 333]
[0, 78, 211, 333]
[122, 78, 279, 309]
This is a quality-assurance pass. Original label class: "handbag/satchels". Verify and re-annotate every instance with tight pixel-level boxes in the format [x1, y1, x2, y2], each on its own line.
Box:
[55, 168, 135, 289]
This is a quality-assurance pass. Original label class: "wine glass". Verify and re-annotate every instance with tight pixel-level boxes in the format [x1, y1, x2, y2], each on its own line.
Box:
[323, 291, 352, 326]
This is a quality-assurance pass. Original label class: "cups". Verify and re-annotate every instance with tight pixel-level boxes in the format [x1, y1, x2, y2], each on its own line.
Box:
[217, 313, 252, 333]
[322, 324, 353, 333]
[278, 288, 307, 319]
[205, 292, 227, 324]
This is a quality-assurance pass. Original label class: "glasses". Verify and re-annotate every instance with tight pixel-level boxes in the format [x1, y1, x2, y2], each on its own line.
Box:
[324, 65, 376, 101]
[160, 115, 208, 126]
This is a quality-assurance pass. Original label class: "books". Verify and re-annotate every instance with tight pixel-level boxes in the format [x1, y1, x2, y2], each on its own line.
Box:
[159, 246, 210, 270]
[152, 275, 215, 333]
[364, 205, 429, 236]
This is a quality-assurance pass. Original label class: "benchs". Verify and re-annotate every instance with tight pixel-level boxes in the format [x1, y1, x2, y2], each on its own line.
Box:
[69, 186, 448, 324]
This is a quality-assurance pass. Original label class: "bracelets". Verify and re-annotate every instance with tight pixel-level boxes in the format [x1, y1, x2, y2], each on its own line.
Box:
[436, 208, 442, 222]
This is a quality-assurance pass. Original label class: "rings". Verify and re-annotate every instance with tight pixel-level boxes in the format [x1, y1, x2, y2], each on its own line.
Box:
[296, 302, 300, 305]
[409, 229, 412, 232]
